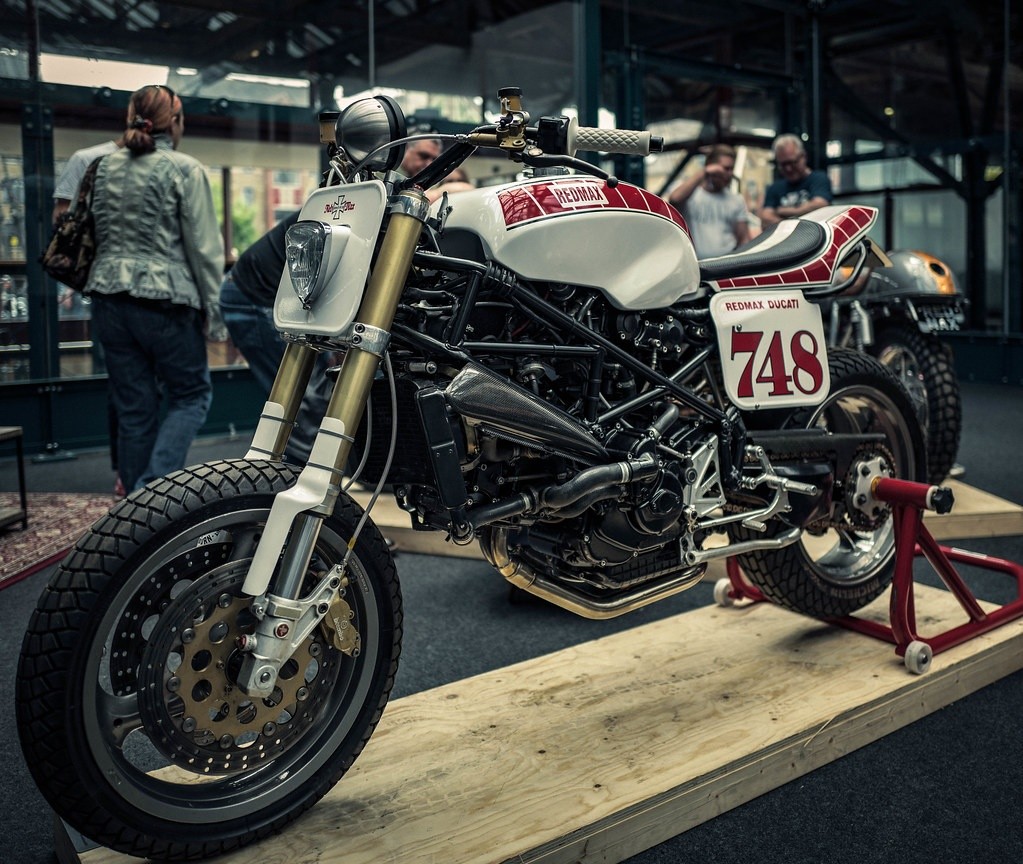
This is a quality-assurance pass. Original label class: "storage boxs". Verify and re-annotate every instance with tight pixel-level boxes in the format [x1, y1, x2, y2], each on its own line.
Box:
[59, 353, 94, 377]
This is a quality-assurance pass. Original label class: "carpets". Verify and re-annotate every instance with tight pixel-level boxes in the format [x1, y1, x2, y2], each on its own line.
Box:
[0, 489, 120, 593]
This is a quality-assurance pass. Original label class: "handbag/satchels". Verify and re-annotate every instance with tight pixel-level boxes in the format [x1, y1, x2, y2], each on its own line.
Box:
[38, 153, 105, 295]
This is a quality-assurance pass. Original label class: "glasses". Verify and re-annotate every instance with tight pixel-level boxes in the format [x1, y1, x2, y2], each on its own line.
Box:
[778, 153, 808, 168]
[142, 85, 174, 109]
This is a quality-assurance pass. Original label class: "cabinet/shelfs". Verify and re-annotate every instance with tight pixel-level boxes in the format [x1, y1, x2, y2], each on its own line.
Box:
[0, 259, 92, 340]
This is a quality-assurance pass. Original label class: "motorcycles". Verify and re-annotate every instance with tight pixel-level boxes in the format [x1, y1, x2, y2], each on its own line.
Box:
[15, 83, 975, 863]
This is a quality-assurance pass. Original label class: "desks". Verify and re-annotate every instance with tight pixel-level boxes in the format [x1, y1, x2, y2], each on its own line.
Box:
[0, 426, 29, 532]
[0, 341, 93, 352]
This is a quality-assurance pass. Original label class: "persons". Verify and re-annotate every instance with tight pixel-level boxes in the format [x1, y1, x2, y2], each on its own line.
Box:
[53, 83, 227, 498]
[220, 121, 474, 437]
[669, 134, 833, 260]
